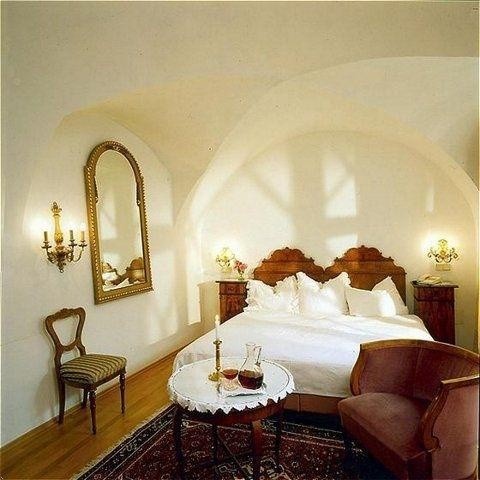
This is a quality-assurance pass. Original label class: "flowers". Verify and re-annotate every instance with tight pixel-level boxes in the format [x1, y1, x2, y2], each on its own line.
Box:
[233, 259, 248, 275]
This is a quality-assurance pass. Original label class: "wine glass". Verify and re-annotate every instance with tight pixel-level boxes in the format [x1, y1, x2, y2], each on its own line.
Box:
[221, 362, 238, 392]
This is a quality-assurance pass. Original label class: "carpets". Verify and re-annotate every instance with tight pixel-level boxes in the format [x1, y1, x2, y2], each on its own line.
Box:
[66, 400, 401, 480]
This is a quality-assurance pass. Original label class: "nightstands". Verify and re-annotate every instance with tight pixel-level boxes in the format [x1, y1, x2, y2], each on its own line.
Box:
[216, 279, 249, 324]
[410, 280, 460, 346]
[171, 244, 436, 431]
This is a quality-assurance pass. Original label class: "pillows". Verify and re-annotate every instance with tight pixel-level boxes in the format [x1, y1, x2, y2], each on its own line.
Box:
[345, 285, 397, 317]
[371, 275, 409, 316]
[243, 275, 298, 322]
[295, 271, 351, 320]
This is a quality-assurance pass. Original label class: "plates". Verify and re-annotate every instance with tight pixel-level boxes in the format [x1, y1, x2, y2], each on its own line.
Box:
[218, 382, 267, 396]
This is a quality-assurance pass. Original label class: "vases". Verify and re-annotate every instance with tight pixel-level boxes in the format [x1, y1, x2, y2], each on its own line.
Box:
[238, 273, 244, 280]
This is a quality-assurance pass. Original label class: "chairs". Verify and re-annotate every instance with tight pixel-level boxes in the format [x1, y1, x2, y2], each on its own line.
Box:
[45, 307, 128, 434]
[337, 339, 480, 480]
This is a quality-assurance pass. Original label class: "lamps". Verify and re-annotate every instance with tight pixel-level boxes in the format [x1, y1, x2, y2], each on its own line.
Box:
[427, 239, 460, 271]
[215, 246, 236, 279]
[39, 200, 87, 275]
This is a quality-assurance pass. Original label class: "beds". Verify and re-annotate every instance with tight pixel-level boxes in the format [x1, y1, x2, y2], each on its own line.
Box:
[101, 256, 147, 292]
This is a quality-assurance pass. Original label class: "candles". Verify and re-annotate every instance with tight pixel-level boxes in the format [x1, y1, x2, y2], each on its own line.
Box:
[215, 315, 220, 341]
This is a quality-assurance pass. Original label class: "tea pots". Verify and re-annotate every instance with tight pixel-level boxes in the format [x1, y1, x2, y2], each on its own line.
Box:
[237, 342, 265, 391]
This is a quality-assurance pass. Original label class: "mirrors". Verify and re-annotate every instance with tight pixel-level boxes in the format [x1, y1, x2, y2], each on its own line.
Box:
[83, 140, 154, 304]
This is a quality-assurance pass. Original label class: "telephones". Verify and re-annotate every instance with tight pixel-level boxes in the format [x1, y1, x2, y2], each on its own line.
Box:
[417, 272, 442, 285]
